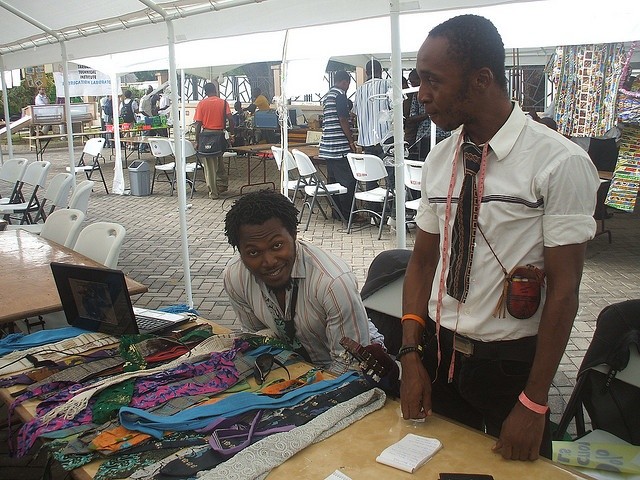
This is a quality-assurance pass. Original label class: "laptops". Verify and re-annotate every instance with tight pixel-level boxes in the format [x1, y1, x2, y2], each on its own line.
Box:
[50, 261, 189, 335]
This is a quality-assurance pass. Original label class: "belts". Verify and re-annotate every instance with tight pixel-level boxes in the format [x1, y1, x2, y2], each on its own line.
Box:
[424, 316, 537, 359]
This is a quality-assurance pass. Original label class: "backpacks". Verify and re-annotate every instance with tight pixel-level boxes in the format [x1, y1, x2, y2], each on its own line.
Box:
[120, 100, 136, 123]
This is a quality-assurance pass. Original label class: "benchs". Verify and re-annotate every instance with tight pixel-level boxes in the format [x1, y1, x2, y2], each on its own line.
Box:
[34, 113, 95, 161]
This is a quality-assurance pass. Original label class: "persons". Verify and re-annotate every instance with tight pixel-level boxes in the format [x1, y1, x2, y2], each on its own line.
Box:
[397, 12, 599, 463]
[100, 95, 113, 148]
[407, 69, 420, 88]
[232, 101, 250, 147]
[34, 84, 52, 136]
[317, 70, 368, 224]
[349, 59, 416, 230]
[193, 82, 236, 201]
[158, 90, 171, 119]
[138, 84, 157, 154]
[408, 88, 452, 160]
[243, 88, 275, 144]
[221, 190, 388, 376]
[118, 90, 140, 151]
[402, 76, 416, 161]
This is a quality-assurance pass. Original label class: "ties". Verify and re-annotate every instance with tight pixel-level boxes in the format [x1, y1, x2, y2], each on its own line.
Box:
[261, 278, 312, 364]
[446, 140, 491, 304]
[0, 304, 266, 460]
[25, 350, 386, 480]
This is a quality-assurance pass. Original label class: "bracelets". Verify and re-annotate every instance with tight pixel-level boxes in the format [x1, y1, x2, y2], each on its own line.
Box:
[400, 313, 426, 331]
[518, 391, 549, 415]
[395, 342, 424, 362]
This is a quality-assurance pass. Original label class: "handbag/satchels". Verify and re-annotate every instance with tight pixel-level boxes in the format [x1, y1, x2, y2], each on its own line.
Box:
[199, 99, 227, 157]
[616, 41, 640, 126]
[461, 143, 547, 319]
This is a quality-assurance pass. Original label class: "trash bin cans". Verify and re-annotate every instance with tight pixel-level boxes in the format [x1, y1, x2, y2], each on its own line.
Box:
[127, 160, 150, 196]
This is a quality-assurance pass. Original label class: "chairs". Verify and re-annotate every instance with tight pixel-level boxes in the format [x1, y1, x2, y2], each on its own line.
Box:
[40, 209, 84, 248]
[65, 138, 109, 194]
[299, 131, 330, 184]
[31, 173, 73, 224]
[72, 221, 127, 270]
[286, 104, 309, 129]
[346, 152, 411, 240]
[292, 148, 353, 231]
[403, 158, 441, 236]
[0, 158, 29, 205]
[0, 161, 51, 224]
[6, 180, 95, 235]
[168, 139, 212, 199]
[221, 152, 241, 175]
[548, 299, 640, 480]
[147, 138, 197, 194]
[360, 252, 450, 355]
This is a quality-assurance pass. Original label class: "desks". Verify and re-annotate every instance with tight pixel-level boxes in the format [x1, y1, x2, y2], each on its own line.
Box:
[226, 140, 319, 186]
[0, 227, 149, 341]
[1, 305, 600, 480]
[259, 146, 332, 198]
[22, 125, 170, 167]
[108, 135, 170, 169]
[339, 150, 624, 244]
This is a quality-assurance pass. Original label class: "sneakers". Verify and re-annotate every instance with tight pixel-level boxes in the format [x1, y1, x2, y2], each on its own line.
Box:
[370, 217, 385, 225]
[344, 213, 367, 221]
[387, 216, 415, 229]
